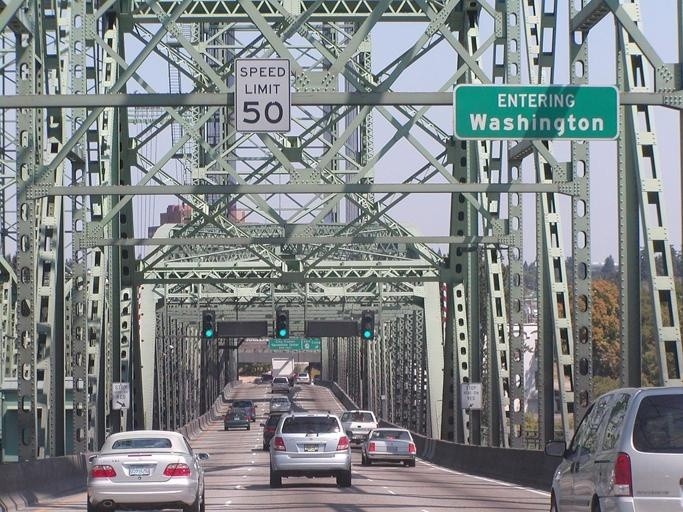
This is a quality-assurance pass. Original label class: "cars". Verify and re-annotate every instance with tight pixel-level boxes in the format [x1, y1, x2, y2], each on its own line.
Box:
[260, 373, 416, 488]
[87, 430, 209, 512]
[222, 400, 257, 431]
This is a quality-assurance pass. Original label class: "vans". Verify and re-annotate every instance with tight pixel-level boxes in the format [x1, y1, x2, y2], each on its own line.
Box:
[544, 387, 683, 512]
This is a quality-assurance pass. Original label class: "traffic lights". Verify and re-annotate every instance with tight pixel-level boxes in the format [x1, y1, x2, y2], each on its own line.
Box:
[203, 310, 215, 339]
[276, 310, 289, 338]
[361, 310, 374, 340]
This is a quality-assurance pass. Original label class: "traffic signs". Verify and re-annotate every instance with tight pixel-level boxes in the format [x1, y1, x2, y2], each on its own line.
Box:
[234, 58, 291, 132]
[268, 338, 321, 351]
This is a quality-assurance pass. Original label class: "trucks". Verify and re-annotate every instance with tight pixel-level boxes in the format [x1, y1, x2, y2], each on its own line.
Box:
[272, 358, 294, 384]
